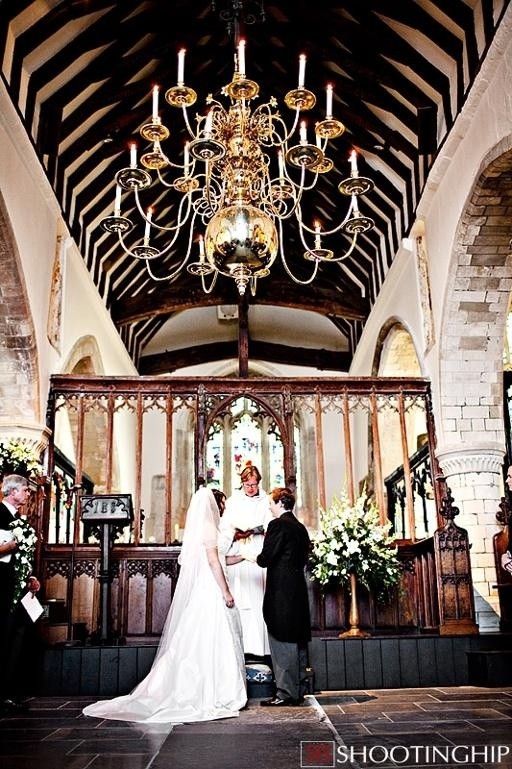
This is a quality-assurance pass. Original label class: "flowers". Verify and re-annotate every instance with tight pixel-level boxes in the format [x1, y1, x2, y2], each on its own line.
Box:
[309, 490, 410, 608]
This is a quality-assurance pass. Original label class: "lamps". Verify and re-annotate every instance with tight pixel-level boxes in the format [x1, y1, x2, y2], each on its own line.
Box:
[99, 1, 378, 297]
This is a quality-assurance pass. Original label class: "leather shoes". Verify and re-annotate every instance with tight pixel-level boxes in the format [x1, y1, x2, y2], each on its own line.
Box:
[259, 696, 305, 706]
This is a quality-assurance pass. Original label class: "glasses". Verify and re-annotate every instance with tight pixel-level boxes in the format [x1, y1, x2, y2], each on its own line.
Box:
[242, 483, 258, 489]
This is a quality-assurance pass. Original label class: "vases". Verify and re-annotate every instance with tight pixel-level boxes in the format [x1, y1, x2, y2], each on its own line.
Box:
[340, 572, 369, 638]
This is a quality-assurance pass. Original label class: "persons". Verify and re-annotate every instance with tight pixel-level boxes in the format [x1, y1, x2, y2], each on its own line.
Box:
[169, 489, 250, 721]
[255, 487, 311, 708]
[218, 465, 277, 706]
[500, 463, 512, 575]
[0, 474, 42, 716]
